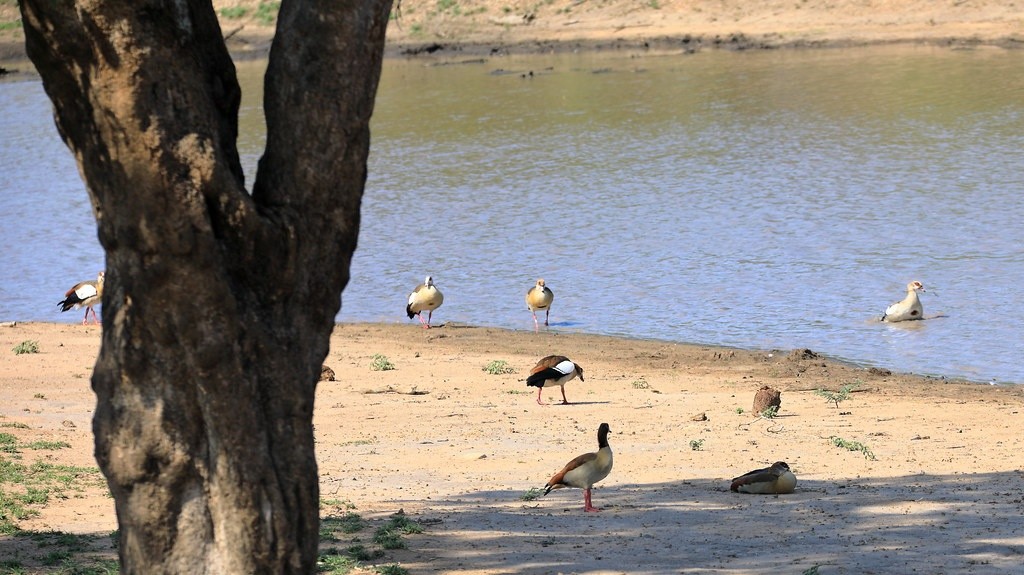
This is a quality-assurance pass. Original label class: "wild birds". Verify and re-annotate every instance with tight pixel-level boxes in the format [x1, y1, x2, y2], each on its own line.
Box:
[525, 279, 554, 324]
[526, 354, 586, 405]
[57, 272, 106, 327]
[406, 275, 444, 330]
[544, 422, 613, 512]
[881, 280, 927, 323]
[730, 461, 799, 494]
